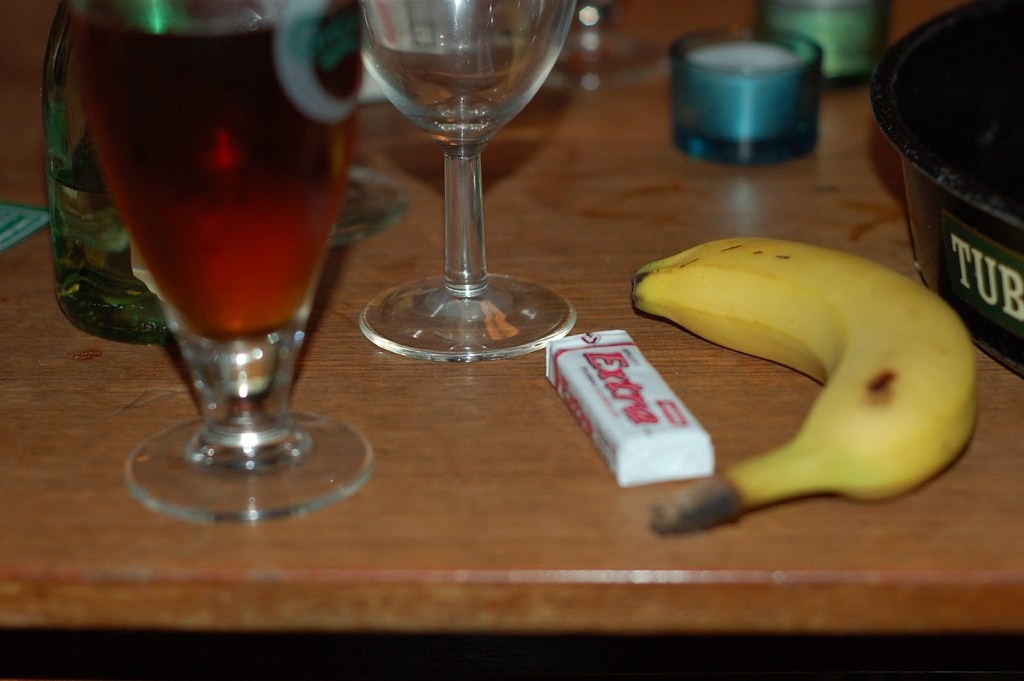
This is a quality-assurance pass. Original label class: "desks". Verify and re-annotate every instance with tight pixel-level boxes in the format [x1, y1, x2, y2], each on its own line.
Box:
[0, 0, 1024, 680]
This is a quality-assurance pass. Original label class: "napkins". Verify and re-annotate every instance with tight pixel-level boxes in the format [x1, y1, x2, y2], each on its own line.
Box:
[547, 330, 716, 488]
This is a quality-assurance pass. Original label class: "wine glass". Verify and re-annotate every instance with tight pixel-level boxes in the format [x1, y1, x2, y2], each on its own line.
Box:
[72, 0, 370, 523]
[360, 0, 577, 360]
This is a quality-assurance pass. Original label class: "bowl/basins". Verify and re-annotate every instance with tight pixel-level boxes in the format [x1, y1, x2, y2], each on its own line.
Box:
[870, 0, 1024, 377]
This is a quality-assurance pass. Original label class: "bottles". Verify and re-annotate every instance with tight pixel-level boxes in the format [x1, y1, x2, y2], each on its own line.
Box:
[41, 0, 176, 346]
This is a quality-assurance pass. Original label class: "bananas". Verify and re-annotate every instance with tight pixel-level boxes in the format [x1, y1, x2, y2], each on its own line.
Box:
[632, 234, 976, 533]
